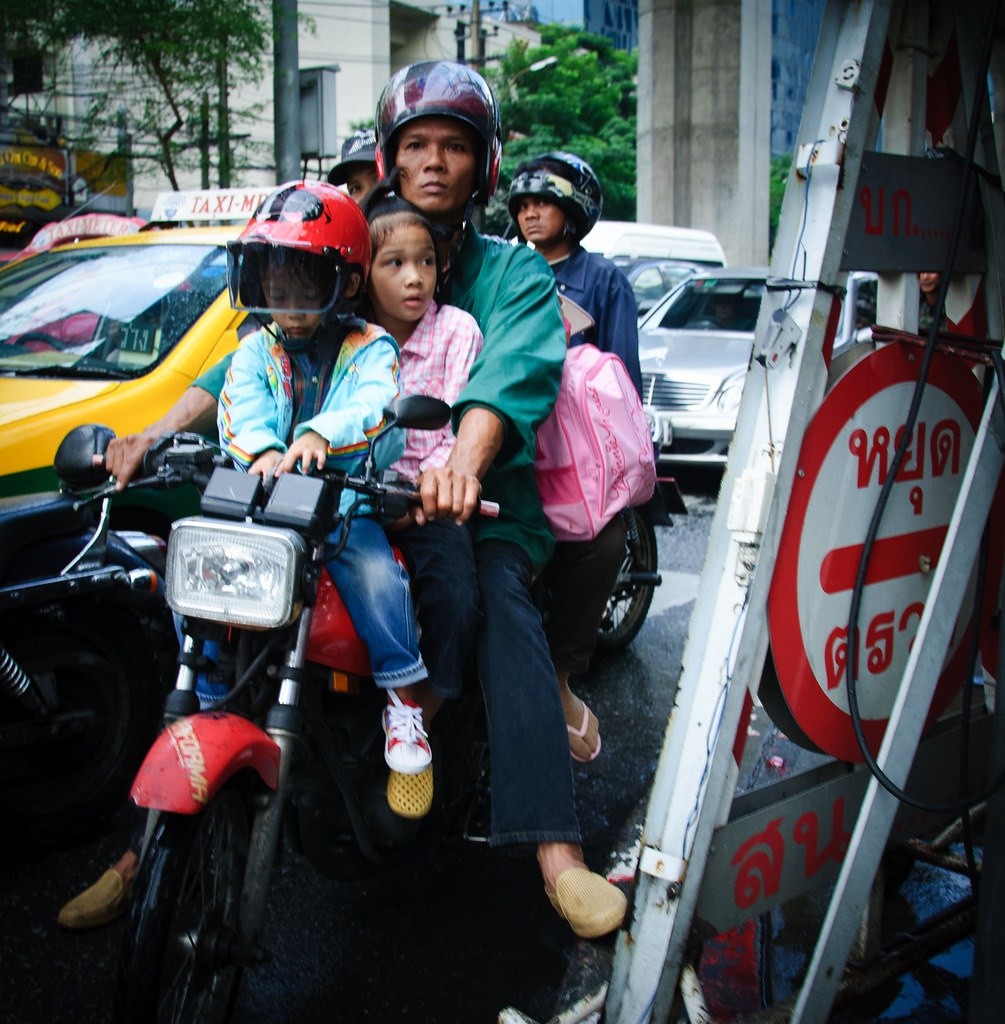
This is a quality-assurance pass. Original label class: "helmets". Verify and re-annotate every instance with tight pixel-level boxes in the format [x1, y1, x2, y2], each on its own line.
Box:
[229, 179, 371, 315]
[508, 151, 603, 245]
[379, 60, 502, 204]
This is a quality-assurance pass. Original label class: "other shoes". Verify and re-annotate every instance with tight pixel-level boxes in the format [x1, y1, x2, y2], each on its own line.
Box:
[542, 871, 628, 941]
[57, 866, 128, 928]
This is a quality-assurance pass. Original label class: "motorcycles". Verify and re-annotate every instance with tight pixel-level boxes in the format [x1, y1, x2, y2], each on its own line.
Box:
[62, 385, 691, 1024]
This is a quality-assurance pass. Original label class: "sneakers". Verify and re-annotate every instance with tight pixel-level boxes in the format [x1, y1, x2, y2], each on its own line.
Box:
[378, 689, 433, 775]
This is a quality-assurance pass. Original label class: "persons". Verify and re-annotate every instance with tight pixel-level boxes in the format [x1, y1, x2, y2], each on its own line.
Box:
[60, 53, 663, 942]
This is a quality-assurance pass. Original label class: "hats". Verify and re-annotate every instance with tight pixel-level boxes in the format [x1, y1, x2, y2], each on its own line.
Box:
[328, 129, 378, 186]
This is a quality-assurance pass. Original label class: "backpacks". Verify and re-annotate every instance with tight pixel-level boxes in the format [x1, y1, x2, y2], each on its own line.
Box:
[535, 345, 657, 543]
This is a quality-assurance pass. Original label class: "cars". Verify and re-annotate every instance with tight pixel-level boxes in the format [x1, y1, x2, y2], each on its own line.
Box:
[0, 179, 285, 855]
[582, 219, 881, 506]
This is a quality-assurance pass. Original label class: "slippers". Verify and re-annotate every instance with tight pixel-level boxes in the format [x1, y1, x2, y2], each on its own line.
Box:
[566, 700, 604, 762]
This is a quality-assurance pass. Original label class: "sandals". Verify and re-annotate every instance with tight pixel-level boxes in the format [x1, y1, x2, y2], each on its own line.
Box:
[387, 767, 434, 818]
